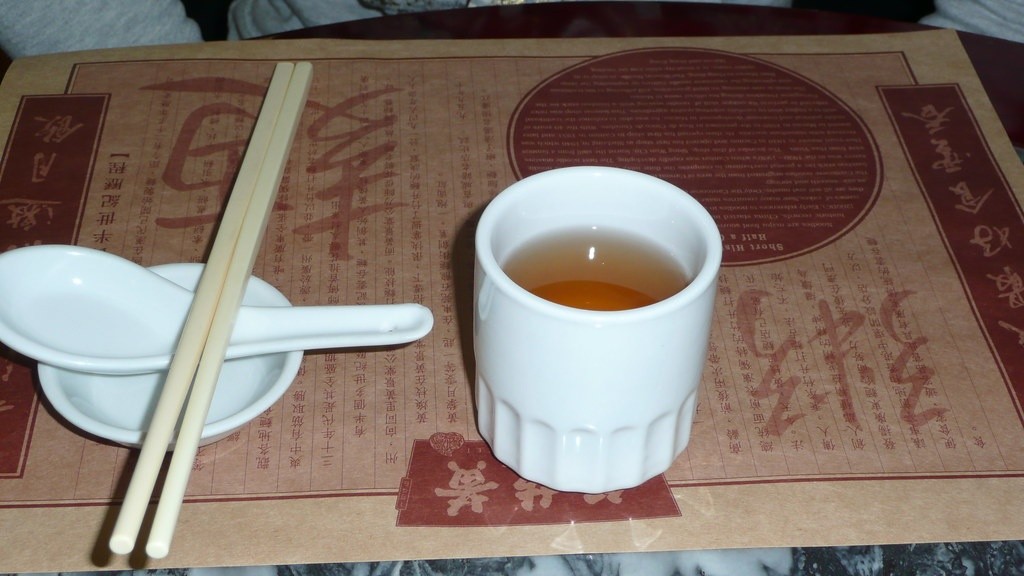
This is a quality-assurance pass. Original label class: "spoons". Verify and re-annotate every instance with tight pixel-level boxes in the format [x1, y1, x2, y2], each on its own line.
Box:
[0, 244, 436, 377]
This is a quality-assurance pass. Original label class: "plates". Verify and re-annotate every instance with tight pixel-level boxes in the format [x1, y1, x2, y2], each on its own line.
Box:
[36, 263, 303, 448]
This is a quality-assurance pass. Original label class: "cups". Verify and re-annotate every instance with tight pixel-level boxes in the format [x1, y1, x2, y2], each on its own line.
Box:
[475, 165, 724, 493]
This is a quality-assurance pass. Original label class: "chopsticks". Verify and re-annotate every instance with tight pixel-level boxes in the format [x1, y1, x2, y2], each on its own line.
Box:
[112, 60, 316, 557]
